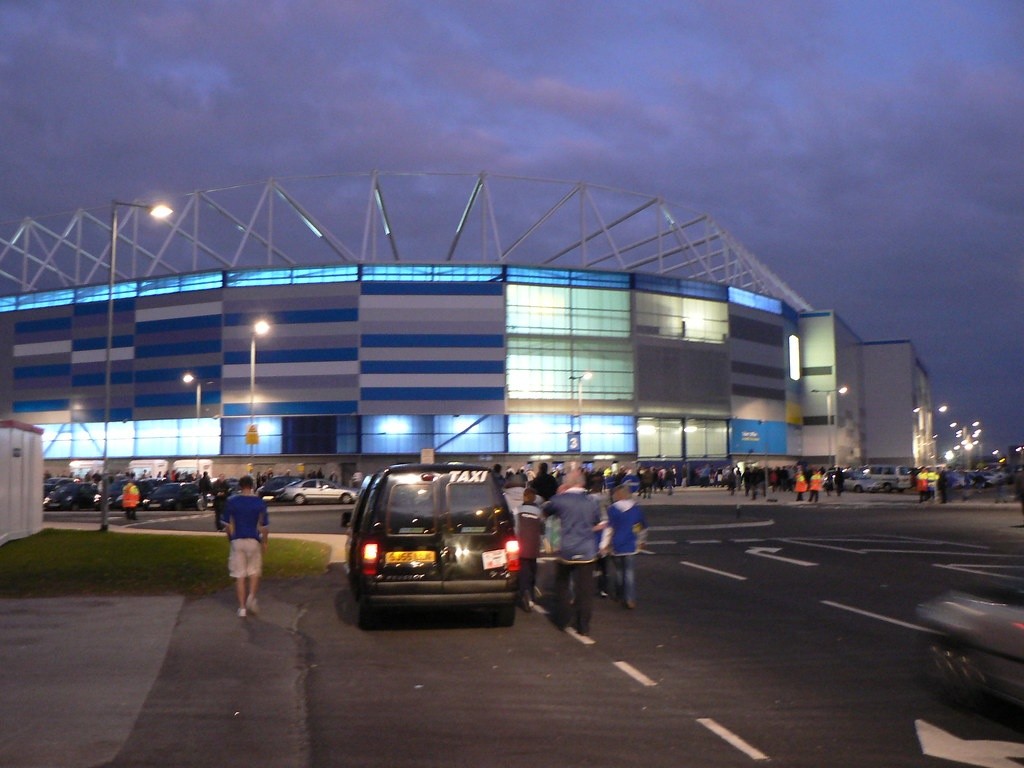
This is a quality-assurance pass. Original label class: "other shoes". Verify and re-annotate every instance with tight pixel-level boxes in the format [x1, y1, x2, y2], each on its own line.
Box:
[245, 592, 258, 613]
[551, 612, 587, 637]
[236, 608, 246, 617]
[625, 599, 635, 608]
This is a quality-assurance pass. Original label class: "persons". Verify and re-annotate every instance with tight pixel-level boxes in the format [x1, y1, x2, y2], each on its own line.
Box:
[637, 463, 851, 503]
[43, 468, 202, 485]
[220, 475, 270, 616]
[123, 480, 140, 521]
[247, 467, 364, 493]
[199, 472, 211, 510]
[912, 466, 1007, 504]
[1014, 467, 1024, 518]
[492, 460, 646, 637]
[211, 474, 228, 533]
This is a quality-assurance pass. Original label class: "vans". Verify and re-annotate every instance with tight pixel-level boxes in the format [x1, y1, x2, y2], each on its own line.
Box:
[341, 462, 521, 629]
[859, 465, 912, 493]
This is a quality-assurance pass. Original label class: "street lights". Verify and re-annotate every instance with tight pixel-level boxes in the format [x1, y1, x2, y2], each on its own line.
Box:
[101, 198, 173, 531]
[578, 372, 591, 431]
[248, 322, 267, 477]
[184, 376, 201, 480]
[913, 406, 982, 460]
[811, 387, 848, 468]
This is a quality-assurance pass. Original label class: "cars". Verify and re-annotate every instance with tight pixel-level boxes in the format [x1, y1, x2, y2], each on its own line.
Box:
[209, 478, 241, 494]
[917, 465, 945, 489]
[255, 476, 304, 503]
[43, 477, 80, 497]
[43, 482, 99, 512]
[92, 479, 155, 510]
[915, 587, 1024, 738]
[142, 482, 206, 512]
[965, 464, 1024, 483]
[275, 478, 359, 505]
[821, 470, 879, 494]
